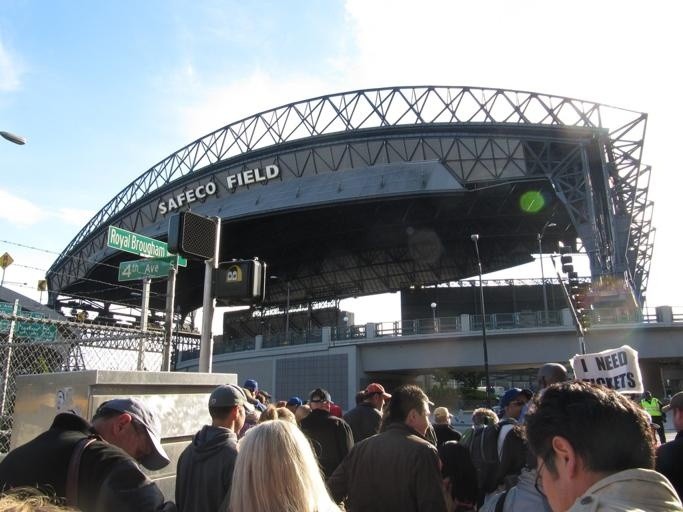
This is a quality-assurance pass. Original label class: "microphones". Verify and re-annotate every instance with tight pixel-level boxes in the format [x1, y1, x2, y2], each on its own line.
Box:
[471, 233, 483, 265]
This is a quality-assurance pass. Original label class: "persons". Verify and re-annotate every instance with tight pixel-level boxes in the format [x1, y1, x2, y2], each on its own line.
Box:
[1, 398, 177, 510]
[176, 363, 682, 512]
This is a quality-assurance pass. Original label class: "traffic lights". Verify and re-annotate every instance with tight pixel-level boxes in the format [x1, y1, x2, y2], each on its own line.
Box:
[561, 245, 573, 273]
[576, 295, 592, 330]
[569, 271, 578, 295]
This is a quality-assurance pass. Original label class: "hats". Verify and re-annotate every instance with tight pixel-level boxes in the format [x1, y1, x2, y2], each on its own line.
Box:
[287, 397, 303, 405]
[661, 391, 683, 413]
[365, 383, 392, 400]
[244, 379, 272, 399]
[97, 398, 171, 471]
[309, 388, 334, 405]
[500, 388, 533, 408]
[208, 383, 257, 414]
[434, 407, 453, 418]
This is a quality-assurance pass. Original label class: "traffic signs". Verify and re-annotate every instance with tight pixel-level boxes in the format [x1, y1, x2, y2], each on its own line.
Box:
[107, 226, 188, 270]
[0, 302, 19, 337]
[0, 280, 27, 288]
[119, 255, 177, 283]
[15, 310, 58, 346]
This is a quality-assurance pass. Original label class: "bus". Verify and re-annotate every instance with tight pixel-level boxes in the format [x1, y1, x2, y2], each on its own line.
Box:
[472, 386, 504, 398]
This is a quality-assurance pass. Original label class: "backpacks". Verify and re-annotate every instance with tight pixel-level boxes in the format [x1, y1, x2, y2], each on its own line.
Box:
[458, 419, 517, 475]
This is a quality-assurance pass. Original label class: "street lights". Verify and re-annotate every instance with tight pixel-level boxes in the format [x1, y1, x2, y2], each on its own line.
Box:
[342, 316, 348, 339]
[468, 232, 494, 419]
[429, 300, 437, 334]
[269, 274, 290, 345]
[536, 217, 561, 326]
